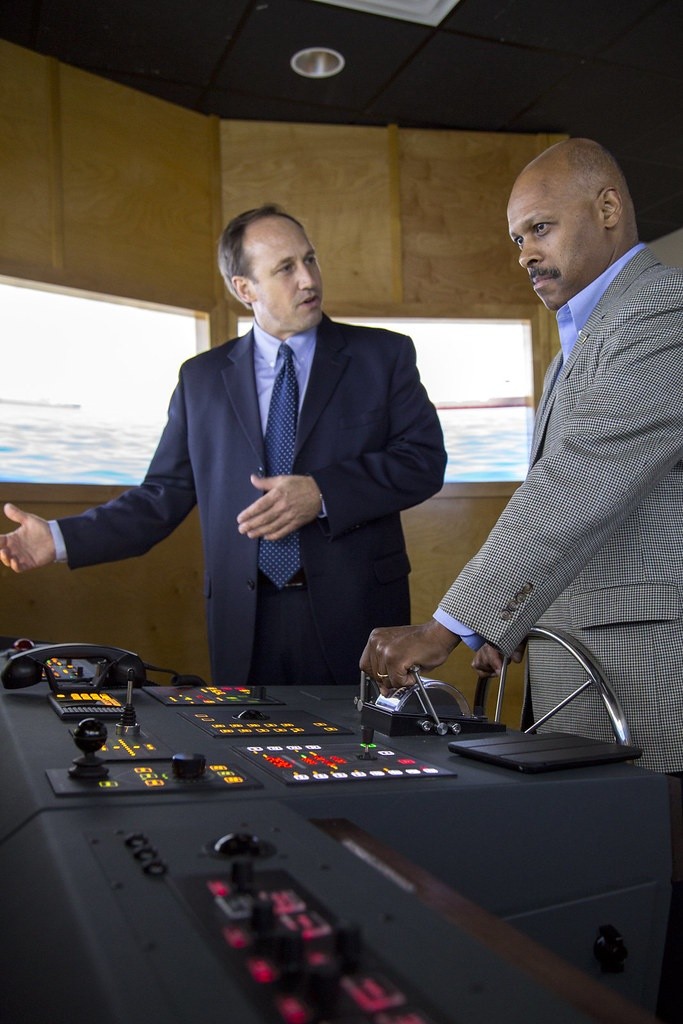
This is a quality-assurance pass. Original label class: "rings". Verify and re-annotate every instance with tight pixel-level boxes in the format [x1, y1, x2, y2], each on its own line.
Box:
[377, 671, 388, 679]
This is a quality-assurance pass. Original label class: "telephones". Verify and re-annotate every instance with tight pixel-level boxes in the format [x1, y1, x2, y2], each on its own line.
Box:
[0, 642, 148, 690]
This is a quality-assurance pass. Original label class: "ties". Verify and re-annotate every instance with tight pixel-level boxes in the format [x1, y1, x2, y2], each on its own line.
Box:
[257, 343, 303, 589]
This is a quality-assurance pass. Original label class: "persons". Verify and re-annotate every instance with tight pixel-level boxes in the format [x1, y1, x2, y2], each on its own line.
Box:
[1, 203, 448, 688]
[361, 139, 683, 781]
